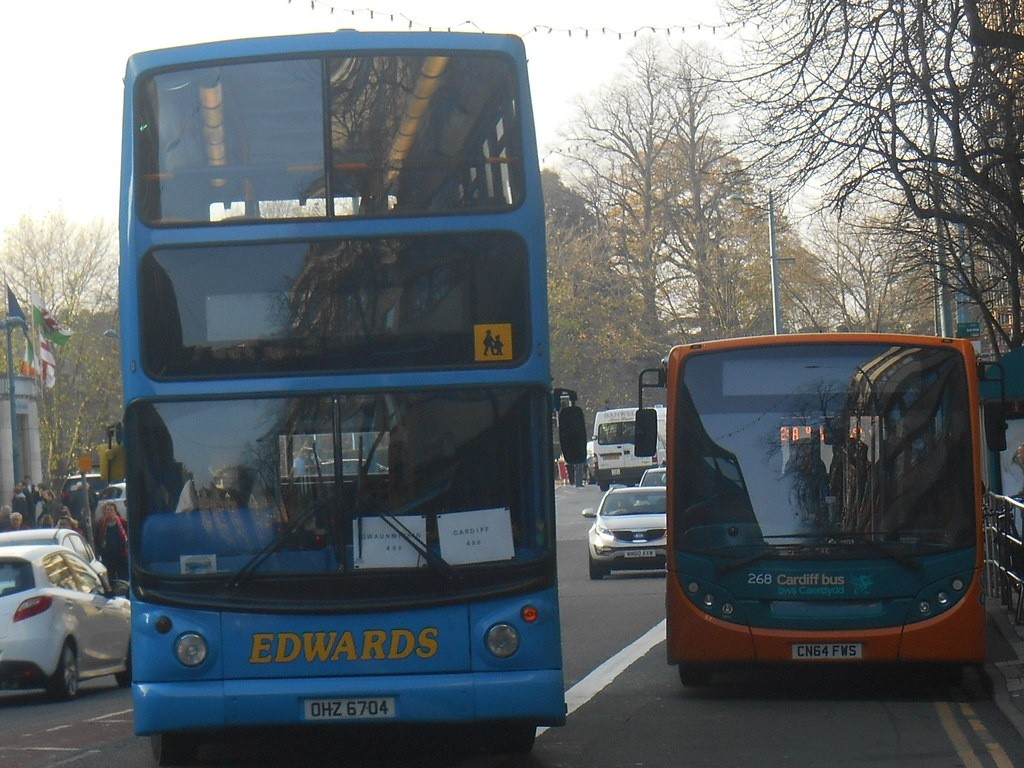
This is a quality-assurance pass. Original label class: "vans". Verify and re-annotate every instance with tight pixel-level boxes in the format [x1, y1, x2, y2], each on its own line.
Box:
[593, 405, 666, 491]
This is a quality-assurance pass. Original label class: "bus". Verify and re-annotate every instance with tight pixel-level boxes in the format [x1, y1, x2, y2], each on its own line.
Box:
[634, 332, 1009, 687]
[100, 28, 587, 768]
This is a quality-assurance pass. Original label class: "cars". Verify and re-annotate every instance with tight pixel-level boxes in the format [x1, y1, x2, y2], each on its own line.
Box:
[60, 472, 127, 531]
[0, 529, 133, 703]
[582, 468, 667, 579]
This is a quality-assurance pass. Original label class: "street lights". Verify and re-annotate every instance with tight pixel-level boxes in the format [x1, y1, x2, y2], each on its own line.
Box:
[1, 315, 24, 486]
[727, 194, 779, 335]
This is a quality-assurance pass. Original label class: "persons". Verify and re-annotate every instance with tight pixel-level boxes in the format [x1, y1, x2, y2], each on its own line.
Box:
[175, 434, 258, 513]
[412, 148, 463, 210]
[0, 476, 128, 585]
[785, 437, 928, 537]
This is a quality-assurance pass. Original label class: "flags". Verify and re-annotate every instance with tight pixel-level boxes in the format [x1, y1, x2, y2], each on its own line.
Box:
[32, 288, 75, 347]
[8, 285, 28, 330]
[40, 333, 55, 389]
[21, 337, 41, 377]
